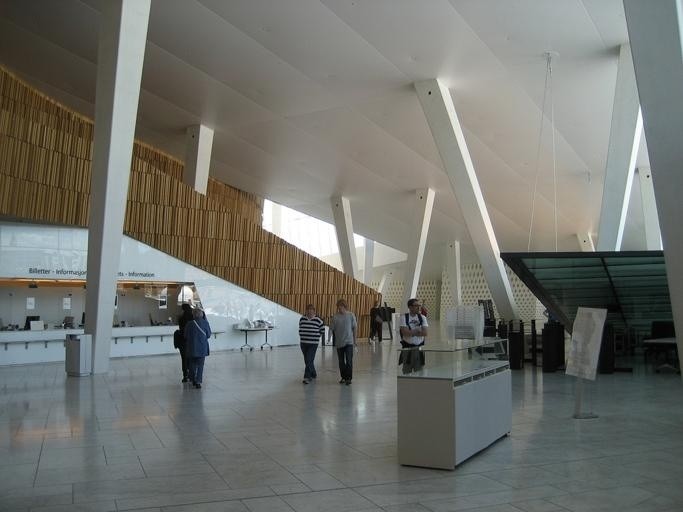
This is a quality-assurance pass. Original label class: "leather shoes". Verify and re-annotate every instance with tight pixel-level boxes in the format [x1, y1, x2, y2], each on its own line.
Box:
[182, 378, 205, 388]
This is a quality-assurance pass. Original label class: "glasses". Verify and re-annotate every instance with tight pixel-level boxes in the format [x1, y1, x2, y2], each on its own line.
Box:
[410, 305, 421, 307]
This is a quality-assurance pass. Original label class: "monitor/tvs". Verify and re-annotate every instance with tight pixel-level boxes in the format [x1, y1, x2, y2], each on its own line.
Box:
[23, 316, 40, 330]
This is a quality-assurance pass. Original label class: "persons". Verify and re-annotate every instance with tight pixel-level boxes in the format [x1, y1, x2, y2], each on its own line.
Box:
[368, 301, 382, 344]
[183, 307, 211, 388]
[298, 304, 324, 383]
[327, 299, 357, 385]
[178, 303, 206, 382]
[399, 298, 428, 374]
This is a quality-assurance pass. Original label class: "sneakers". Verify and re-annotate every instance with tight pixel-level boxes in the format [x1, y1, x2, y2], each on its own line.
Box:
[339, 378, 345, 383]
[346, 379, 350, 384]
[302, 377, 311, 383]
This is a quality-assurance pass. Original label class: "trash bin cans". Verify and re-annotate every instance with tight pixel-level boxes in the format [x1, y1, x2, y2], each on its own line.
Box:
[65, 333, 92, 376]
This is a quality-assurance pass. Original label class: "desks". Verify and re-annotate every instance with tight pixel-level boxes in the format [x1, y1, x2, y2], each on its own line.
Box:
[234, 326, 274, 352]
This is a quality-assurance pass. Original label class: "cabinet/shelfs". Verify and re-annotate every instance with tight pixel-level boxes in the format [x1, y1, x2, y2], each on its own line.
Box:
[394, 335, 513, 473]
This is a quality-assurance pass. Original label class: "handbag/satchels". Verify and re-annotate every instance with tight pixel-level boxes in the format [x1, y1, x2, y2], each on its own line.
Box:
[174, 329, 181, 349]
[206, 342, 209, 356]
[376, 315, 383, 324]
